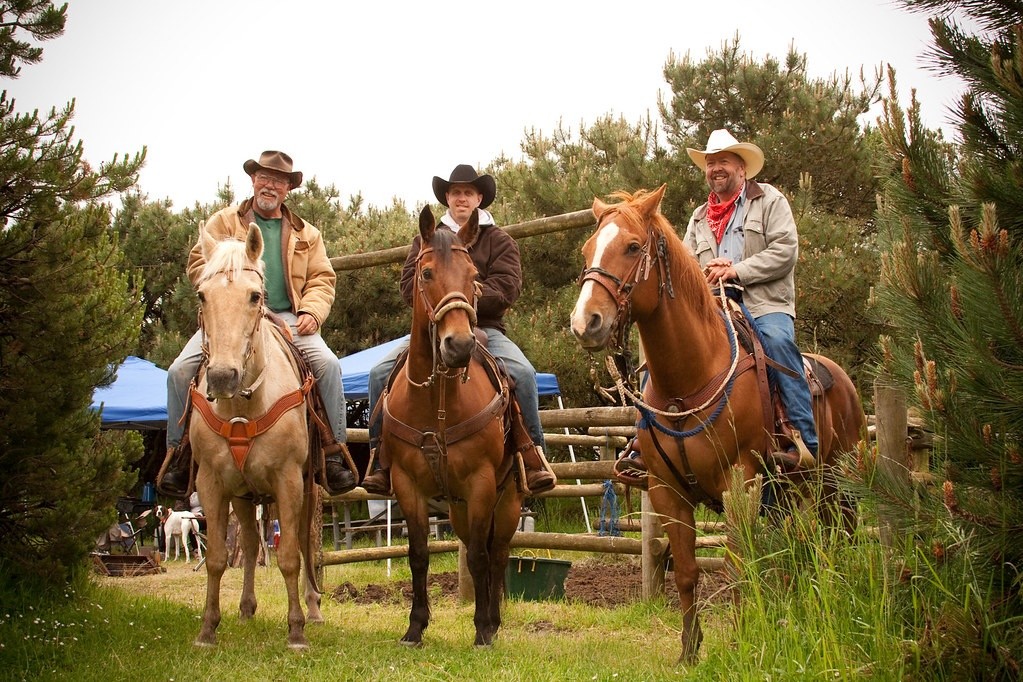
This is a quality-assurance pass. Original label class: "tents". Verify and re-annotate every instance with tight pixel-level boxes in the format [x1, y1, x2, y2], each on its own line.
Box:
[91, 355, 172, 431]
[336, 331, 591, 580]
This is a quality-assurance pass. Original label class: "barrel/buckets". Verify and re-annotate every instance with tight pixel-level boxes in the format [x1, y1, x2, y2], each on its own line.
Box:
[503, 547, 574, 604]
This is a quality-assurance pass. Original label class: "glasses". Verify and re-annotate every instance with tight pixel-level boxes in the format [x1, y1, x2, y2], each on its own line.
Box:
[254, 174, 291, 190]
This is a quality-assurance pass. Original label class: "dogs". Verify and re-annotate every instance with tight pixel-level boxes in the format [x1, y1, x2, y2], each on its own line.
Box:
[153, 504, 207, 564]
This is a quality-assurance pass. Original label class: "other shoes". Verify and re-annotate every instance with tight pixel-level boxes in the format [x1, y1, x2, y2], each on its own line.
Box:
[361, 468, 391, 493]
[618, 456, 646, 477]
[516, 468, 553, 492]
[315, 460, 354, 490]
[770, 450, 800, 467]
[163, 471, 191, 493]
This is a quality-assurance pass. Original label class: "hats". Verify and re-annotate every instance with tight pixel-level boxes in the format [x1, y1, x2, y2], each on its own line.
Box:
[243, 151, 303, 190]
[687, 129, 765, 180]
[432, 164, 495, 209]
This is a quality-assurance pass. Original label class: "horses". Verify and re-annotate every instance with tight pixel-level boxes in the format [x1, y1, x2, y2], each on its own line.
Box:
[570, 182, 872, 663]
[380, 204, 532, 653]
[186, 219, 319, 652]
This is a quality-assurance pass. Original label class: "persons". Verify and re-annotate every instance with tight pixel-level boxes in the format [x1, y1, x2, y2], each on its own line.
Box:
[616, 129, 820, 475]
[361, 165, 554, 497]
[159, 151, 356, 493]
